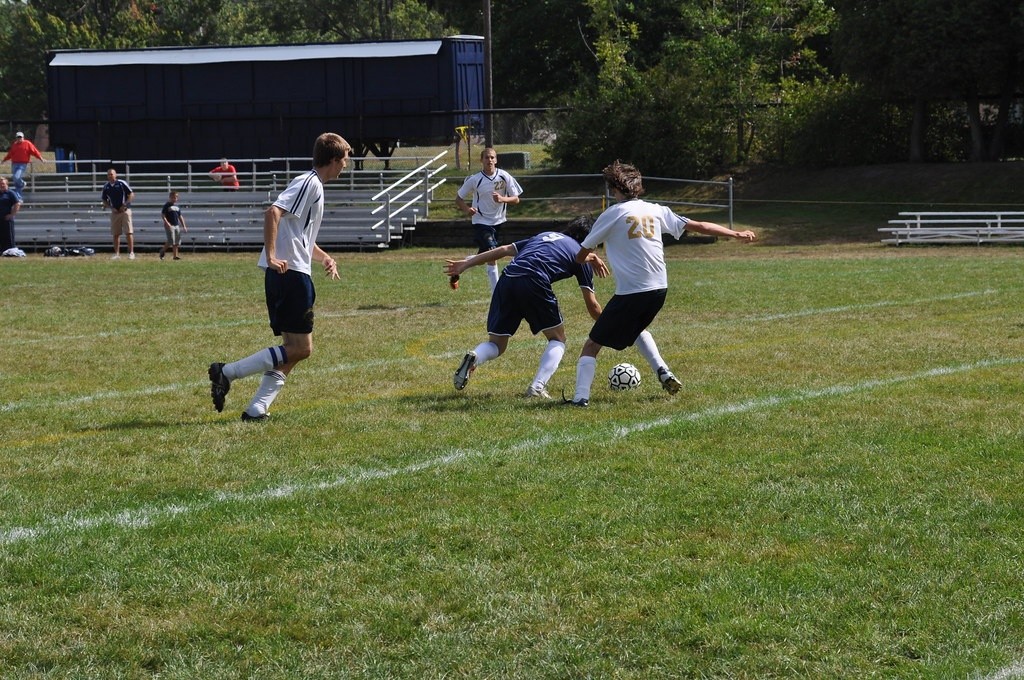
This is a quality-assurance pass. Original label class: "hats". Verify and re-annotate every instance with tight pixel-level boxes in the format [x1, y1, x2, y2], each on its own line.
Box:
[16, 132, 24, 138]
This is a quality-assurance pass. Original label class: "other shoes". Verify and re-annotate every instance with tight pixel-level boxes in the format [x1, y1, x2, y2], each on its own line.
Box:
[129, 252, 136, 260]
[159, 249, 181, 260]
[110, 255, 121, 260]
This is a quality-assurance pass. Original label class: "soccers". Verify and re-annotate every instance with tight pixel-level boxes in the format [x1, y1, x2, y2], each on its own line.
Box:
[607, 363, 642, 392]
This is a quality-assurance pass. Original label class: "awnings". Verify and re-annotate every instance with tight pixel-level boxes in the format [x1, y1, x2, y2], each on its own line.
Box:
[48, 40, 442, 67]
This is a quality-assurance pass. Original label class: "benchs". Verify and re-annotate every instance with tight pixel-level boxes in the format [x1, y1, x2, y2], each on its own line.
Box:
[878, 212, 1024, 248]
[11, 189, 434, 252]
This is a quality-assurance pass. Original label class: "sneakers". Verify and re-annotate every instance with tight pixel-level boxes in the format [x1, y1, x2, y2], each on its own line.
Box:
[450, 273, 460, 291]
[525, 386, 552, 400]
[453, 351, 478, 392]
[561, 398, 591, 409]
[242, 411, 270, 422]
[659, 369, 681, 394]
[208, 362, 231, 414]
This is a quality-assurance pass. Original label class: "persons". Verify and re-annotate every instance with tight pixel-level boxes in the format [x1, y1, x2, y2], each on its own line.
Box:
[575, 163, 756, 407]
[208, 157, 240, 192]
[158, 191, 188, 261]
[207, 132, 352, 424]
[441, 214, 604, 402]
[455, 147, 525, 303]
[0, 176, 22, 258]
[101, 168, 135, 260]
[0, 131, 47, 193]
